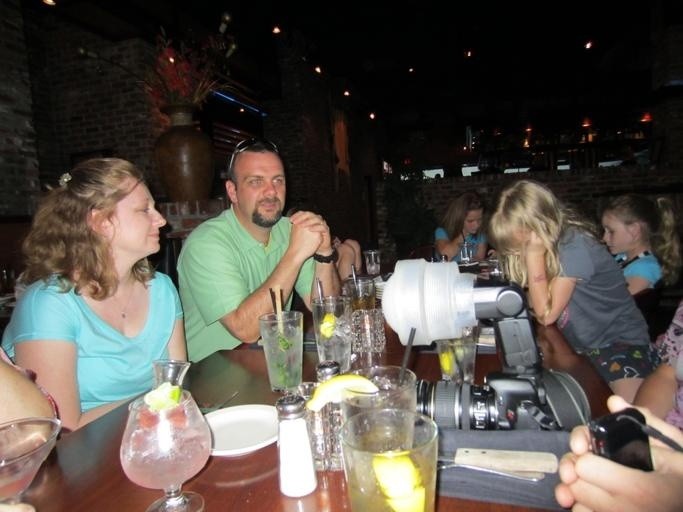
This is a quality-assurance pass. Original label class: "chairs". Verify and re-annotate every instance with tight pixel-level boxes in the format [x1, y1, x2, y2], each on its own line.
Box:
[631, 280, 663, 341]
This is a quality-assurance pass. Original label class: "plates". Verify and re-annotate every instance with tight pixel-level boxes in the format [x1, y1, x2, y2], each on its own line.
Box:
[196, 401, 279, 456]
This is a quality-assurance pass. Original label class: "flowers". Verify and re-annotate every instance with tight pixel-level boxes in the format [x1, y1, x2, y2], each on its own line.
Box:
[136, 35, 231, 103]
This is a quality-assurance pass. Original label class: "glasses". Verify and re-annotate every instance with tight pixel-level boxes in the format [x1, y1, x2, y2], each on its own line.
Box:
[229, 137, 280, 169]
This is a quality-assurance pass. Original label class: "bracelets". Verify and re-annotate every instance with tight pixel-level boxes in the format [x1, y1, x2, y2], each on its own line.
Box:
[111, 275, 141, 323]
[308, 249, 342, 266]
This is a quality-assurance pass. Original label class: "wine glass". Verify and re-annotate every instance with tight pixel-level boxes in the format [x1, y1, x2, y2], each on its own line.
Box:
[0, 417, 64, 507]
[118, 388, 211, 510]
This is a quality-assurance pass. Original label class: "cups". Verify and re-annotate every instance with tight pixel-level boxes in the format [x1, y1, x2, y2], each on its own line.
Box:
[256, 241, 386, 392]
[430, 317, 480, 381]
[337, 409, 442, 512]
[342, 365, 418, 448]
[152, 359, 192, 388]
[457, 242, 474, 262]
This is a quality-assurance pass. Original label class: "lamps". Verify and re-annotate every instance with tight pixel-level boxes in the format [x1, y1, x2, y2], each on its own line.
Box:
[522, 108, 650, 148]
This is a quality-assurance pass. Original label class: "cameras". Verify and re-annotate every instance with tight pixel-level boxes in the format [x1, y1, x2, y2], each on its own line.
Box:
[588, 408, 652, 472]
[415, 281, 560, 432]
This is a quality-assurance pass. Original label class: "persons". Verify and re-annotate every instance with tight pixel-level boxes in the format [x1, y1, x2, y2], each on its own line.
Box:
[552, 299, 683, 512]
[289, 197, 362, 281]
[430, 189, 487, 262]
[0, 158, 188, 441]
[596, 191, 683, 308]
[487, 179, 652, 406]
[174, 136, 339, 366]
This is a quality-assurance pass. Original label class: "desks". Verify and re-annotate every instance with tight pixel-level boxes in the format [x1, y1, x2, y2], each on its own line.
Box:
[21, 261, 614, 511]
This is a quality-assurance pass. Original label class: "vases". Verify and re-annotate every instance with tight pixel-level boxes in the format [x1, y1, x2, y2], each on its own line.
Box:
[153, 103, 216, 214]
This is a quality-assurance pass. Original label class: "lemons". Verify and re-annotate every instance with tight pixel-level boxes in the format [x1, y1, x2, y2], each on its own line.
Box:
[372, 448, 426, 512]
[319, 312, 337, 338]
[439, 351, 454, 376]
[144, 381, 180, 408]
[305, 374, 379, 412]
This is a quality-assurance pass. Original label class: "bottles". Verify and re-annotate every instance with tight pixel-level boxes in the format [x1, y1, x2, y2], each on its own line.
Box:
[273, 360, 343, 498]
